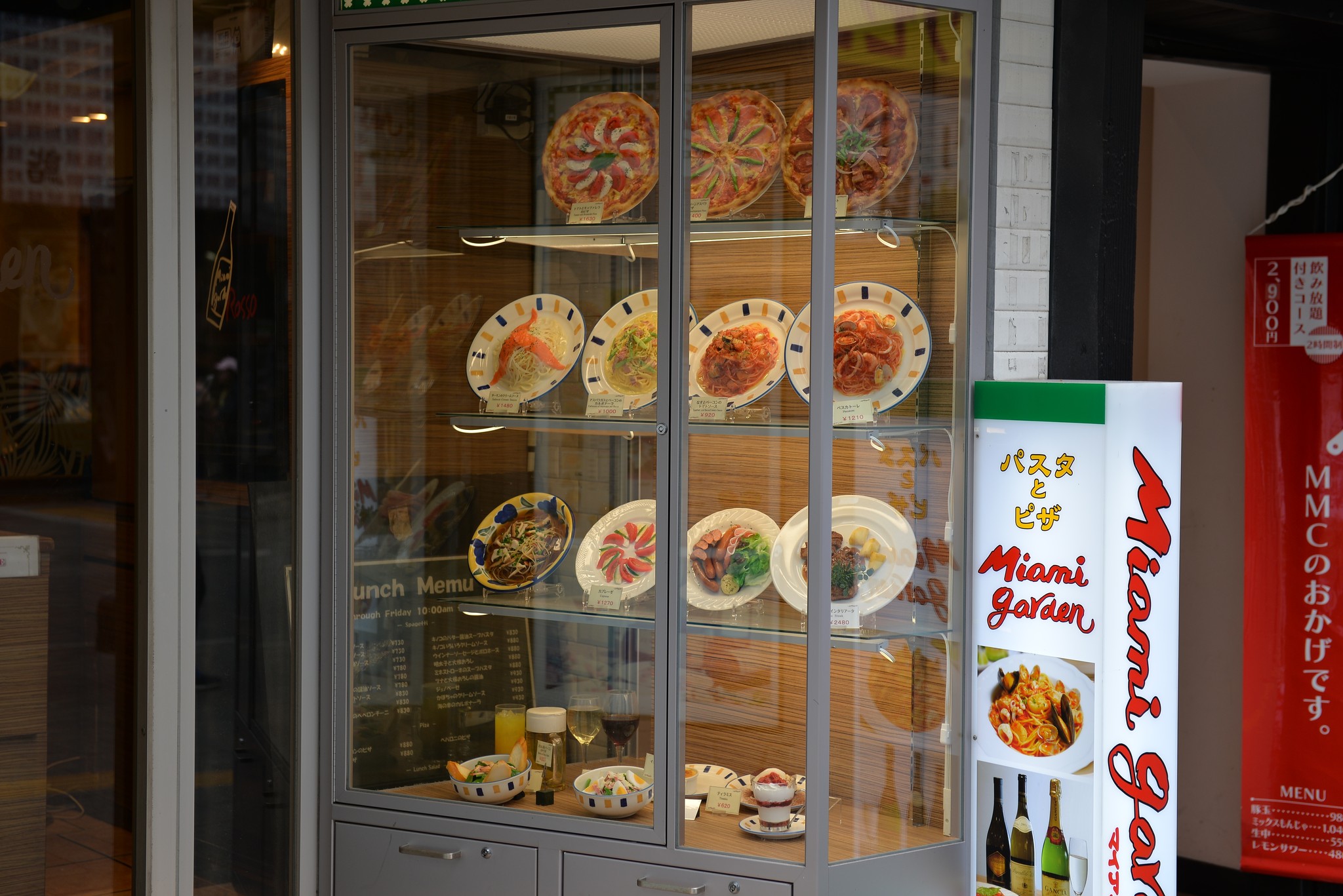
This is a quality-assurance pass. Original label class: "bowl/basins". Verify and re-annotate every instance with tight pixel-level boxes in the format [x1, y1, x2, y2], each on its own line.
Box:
[449, 753, 532, 805]
[572, 766, 653, 819]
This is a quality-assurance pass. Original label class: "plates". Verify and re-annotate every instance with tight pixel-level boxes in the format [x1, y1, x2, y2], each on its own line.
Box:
[467, 492, 575, 594]
[687, 507, 780, 611]
[690, 89, 788, 219]
[542, 91, 659, 220]
[575, 499, 655, 602]
[783, 281, 932, 417]
[465, 293, 587, 405]
[739, 813, 806, 840]
[725, 773, 806, 812]
[685, 764, 739, 800]
[976, 653, 1094, 776]
[976, 882, 1019, 896]
[689, 298, 797, 413]
[580, 287, 699, 412]
[769, 494, 917, 617]
[779, 78, 918, 214]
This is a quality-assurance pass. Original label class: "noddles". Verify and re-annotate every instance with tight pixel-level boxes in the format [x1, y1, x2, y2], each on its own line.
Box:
[486, 513, 564, 587]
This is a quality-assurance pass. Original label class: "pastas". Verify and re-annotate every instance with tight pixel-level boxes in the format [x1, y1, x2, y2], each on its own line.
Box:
[604, 312, 658, 393]
[990, 683, 1082, 757]
[491, 323, 564, 392]
[833, 311, 903, 395]
[696, 322, 779, 397]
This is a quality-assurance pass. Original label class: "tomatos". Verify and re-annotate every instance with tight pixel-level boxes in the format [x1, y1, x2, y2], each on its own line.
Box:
[596, 522, 656, 582]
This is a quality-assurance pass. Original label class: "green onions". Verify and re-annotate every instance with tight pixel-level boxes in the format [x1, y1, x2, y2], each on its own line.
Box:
[496, 517, 551, 567]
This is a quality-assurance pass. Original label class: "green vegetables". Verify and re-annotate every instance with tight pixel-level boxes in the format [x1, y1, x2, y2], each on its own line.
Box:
[465, 761, 522, 783]
[585, 773, 635, 795]
[1015, 676, 1061, 722]
[607, 327, 658, 374]
[831, 560, 874, 597]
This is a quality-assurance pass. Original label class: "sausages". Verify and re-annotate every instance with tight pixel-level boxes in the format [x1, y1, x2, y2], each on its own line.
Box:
[690, 524, 741, 591]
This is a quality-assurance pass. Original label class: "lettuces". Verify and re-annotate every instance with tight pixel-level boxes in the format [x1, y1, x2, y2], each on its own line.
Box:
[725, 533, 771, 587]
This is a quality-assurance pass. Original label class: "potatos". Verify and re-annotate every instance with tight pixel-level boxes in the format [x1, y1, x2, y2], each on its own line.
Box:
[848, 527, 886, 571]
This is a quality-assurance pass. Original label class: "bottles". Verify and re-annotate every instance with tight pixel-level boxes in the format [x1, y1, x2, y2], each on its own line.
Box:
[986, 777, 1011, 891]
[1041, 779, 1070, 896]
[1009, 773, 1036, 896]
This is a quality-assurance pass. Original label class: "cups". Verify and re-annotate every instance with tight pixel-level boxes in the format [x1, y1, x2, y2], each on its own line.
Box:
[1067, 837, 1088, 896]
[525, 706, 567, 792]
[685, 767, 699, 795]
[751, 774, 806, 832]
[494, 703, 526, 755]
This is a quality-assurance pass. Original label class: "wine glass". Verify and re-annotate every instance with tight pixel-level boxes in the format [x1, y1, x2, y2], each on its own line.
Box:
[566, 694, 603, 775]
[601, 688, 640, 766]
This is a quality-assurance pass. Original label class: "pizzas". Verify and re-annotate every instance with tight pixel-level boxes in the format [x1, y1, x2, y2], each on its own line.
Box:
[541, 92, 659, 219]
[780, 77, 917, 215]
[689, 89, 786, 219]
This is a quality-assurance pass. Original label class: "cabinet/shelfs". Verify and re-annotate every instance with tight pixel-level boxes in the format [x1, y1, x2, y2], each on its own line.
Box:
[296, 0, 976, 896]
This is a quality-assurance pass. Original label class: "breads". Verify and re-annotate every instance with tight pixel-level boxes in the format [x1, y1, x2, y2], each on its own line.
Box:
[446, 761, 471, 782]
[481, 760, 511, 783]
[509, 736, 527, 772]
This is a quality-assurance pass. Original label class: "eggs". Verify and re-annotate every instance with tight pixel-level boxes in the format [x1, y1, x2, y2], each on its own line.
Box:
[626, 770, 651, 789]
[582, 776, 605, 795]
[612, 780, 628, 795]
